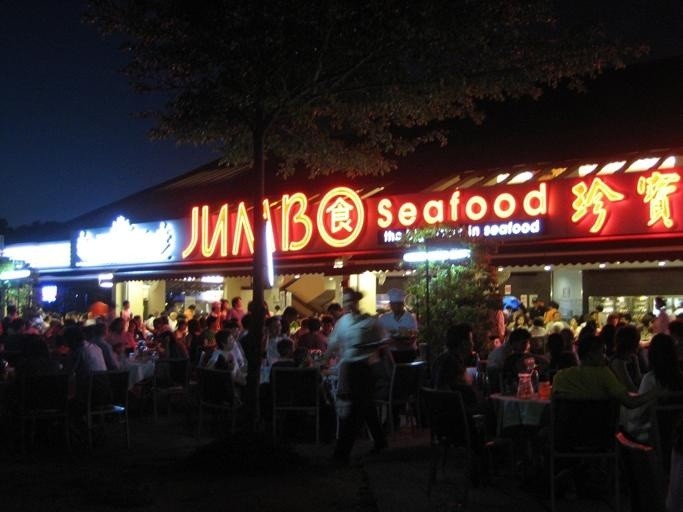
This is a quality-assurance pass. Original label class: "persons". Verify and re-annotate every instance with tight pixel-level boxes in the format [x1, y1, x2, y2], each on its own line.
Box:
[1, 288, 682, 511]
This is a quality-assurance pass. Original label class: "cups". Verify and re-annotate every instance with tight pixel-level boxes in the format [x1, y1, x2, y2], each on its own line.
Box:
[466, 367, 477, 376]
[398, 326, 416, 337]
[129, 352, 134, 361]
[538, 381, 552, 401]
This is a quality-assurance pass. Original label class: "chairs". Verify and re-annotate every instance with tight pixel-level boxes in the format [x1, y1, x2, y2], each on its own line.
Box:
[1, 321, 682, 511]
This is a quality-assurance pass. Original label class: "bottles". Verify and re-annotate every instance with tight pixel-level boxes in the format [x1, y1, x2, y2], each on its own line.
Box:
[525, 358, 539, 392]
[151, 351, 159, 369]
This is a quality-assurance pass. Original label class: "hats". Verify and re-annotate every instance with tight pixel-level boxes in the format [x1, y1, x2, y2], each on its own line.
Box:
[342, 292, 362, 301]
[388, 288, 405, 303]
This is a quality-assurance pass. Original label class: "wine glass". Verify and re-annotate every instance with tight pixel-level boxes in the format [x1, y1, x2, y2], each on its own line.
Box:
[642, 327, 649, 341]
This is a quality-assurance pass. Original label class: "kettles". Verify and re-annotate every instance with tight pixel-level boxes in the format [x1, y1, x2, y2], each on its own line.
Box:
[516, 373, 533, 400]
